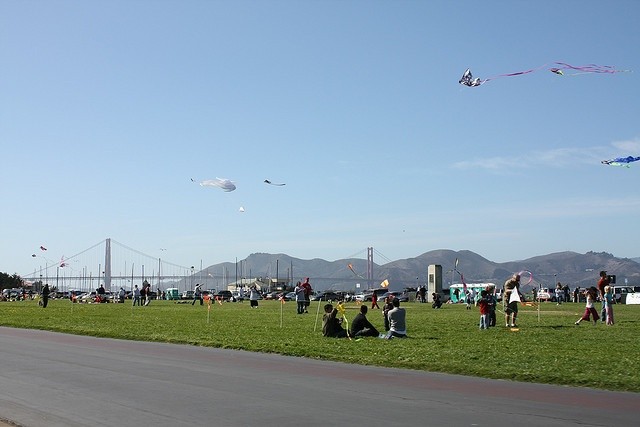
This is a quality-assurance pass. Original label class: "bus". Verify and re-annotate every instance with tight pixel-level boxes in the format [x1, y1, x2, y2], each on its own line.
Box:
[449, 282, 496, 304]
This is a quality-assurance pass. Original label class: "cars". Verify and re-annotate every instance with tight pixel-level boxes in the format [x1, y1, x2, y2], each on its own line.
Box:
[310, 291, 340, 301]
[241, 289, 296, 302]
[377, 290, 410, 302]
[0, 286, 211, 301]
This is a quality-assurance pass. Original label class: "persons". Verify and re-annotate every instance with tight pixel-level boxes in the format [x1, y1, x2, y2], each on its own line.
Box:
[576, 287, 600, 325]
[554, 282, 563, 306]
[572, 285, 580, 303]
[468, 288, 478, 305]
[237, 286, 245, 301]
[465, 291, 470, 310]
[604, 286, 615, 325]
[343, 293, 357, 302]
[302, 277, 313, 306]
[388, 298, 406, 339]
[598, 272, 607, 321]
[562, 283, 571, 302]
[487, 286, 498, 325]
[42, 284, 51, 307]
[533, 287, 537, 302]
[382, 297, 393, 331]
[249, 284, 261, 308]
[1, 289, 32, 303]
[476, 290, 492, 329]
[348, 305, 387, 338]
[294, 282, 304, 311]
[70, 280, 167, 306]
[430, 292, 443, 310]
[191, 283, 204, 306]
[505, 274, 520, 328]
[322, 304, 350, 338]
[415, 282, 425, 299]
[372, 290, 378, 309]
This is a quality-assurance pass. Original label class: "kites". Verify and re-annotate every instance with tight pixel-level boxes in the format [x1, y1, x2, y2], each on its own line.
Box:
[238, 206, 245, 213]
[335, 302, 352, 341]
[39, 246, 47, 250]
[347, 263, 365, 278]
[459, 68, 541, 87]
[509, 286, 524, 304]
[453, 259, 467, 292]
[191, 177, 236, 192]
[59, 255, 77, 269]
[33, 253, 37, 258]
[551, 61, 620, 77]
[380, 279, 391, 288]
[264, 179, 287, 189]
[602, 154, 636, 168]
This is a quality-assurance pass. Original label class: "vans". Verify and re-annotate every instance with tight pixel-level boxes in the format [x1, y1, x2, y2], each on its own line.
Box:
[610, 286, 636, 304]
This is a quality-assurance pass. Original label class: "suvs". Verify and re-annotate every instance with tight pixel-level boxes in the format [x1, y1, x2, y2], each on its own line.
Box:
[536, 287, 557, 302]
[354, 287, 388, 302]
[495, 289, 505, 302]
[211, 290, 232, 299]
